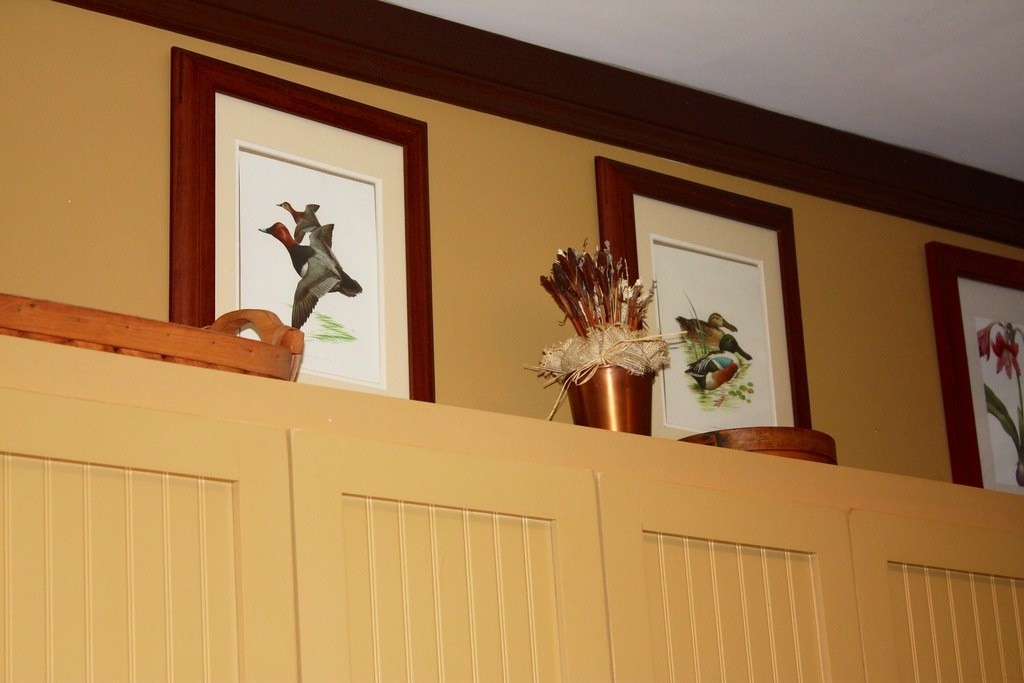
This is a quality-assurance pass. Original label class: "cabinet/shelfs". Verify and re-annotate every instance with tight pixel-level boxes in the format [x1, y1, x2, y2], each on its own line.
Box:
[0, 334, 1024, 683]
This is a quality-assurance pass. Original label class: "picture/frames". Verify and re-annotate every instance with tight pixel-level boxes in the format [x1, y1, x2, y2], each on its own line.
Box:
[592, 153, 814, 441]
[168, 42, 438, 403]
[925, 239, 1024, 491]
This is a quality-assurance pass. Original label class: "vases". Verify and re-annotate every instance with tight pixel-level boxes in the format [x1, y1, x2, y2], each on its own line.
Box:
[561, 364, 655, 438]
